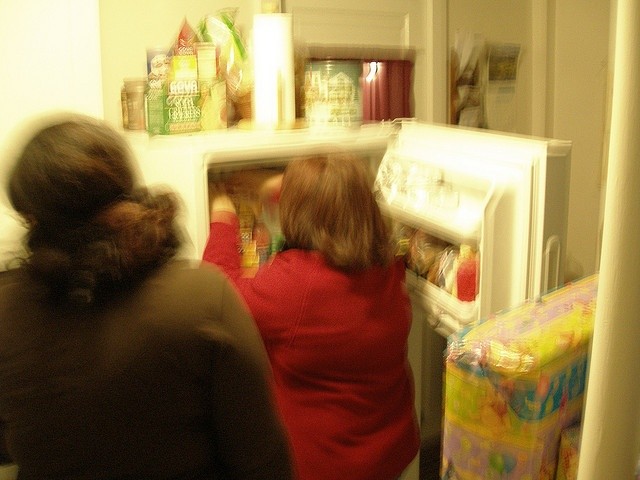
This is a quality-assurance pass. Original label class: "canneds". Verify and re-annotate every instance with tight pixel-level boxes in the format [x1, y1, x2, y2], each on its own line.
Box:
[120, 80, 148, 130]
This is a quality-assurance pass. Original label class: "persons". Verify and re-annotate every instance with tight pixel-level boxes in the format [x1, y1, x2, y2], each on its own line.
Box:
[0, 107, 300, 478]
[200, 155, 421, 479]
